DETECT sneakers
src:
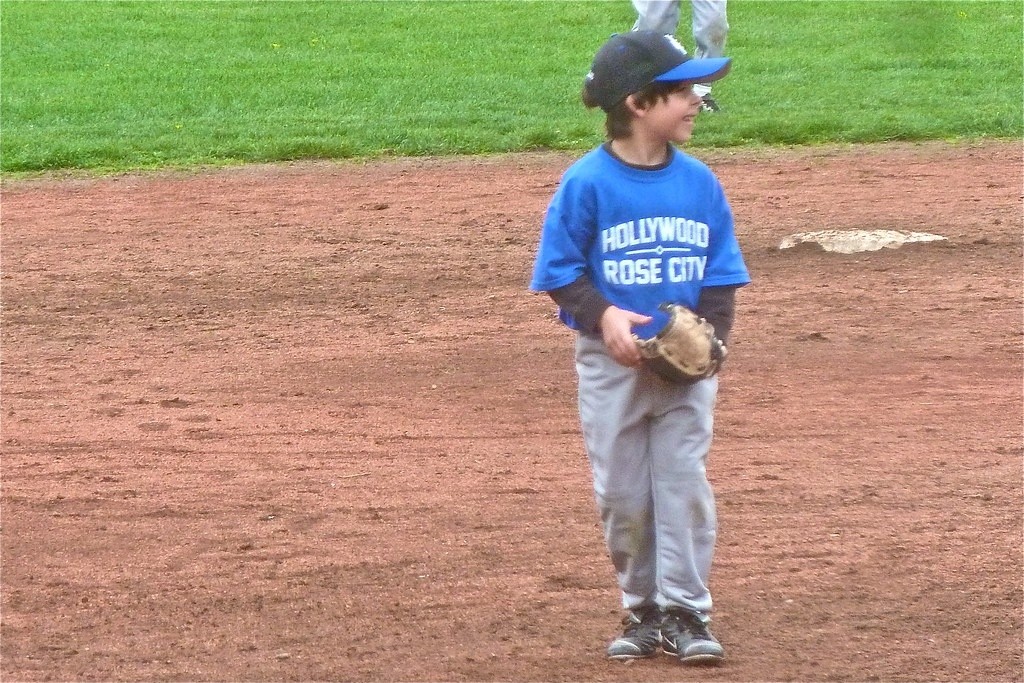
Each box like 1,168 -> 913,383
661,610 -> 725,663
582,88 -> 600,107
606,606 -> 662,660
700,93 -> 721,112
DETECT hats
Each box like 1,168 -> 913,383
586,31 -> 732,113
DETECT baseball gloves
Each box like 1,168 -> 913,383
633,303 -> 724,388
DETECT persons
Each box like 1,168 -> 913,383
531,32 -> 753,663
632,0 -> 729,113
